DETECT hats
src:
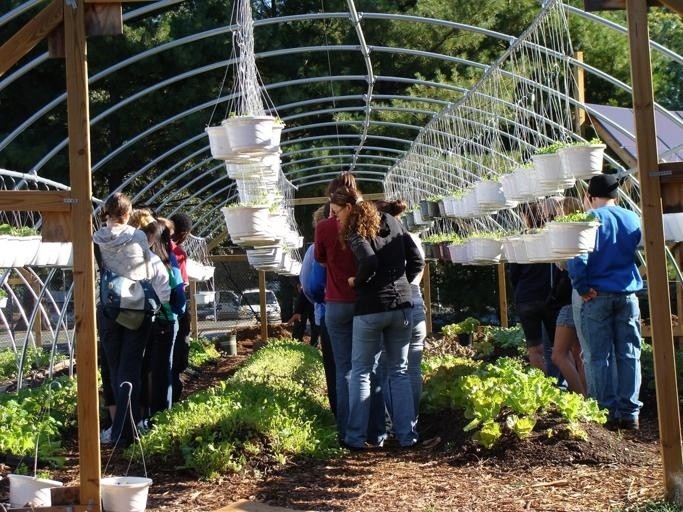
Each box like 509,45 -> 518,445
589,174 -> 618,200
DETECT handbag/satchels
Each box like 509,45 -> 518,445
100,265 -> 157,332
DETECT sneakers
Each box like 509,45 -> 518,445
100,425 -> 112,445
603,415 -> 639,430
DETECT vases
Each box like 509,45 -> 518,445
101,477 -> 152,512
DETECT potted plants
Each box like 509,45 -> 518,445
205,113 -> 304,278
7,469 -> 63,509
0,224 -> 216,282
401,140 -> 607,267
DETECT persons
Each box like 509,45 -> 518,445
506,171 -> 645,431
286,173 -> 444,452
91,190 -> 195,452
